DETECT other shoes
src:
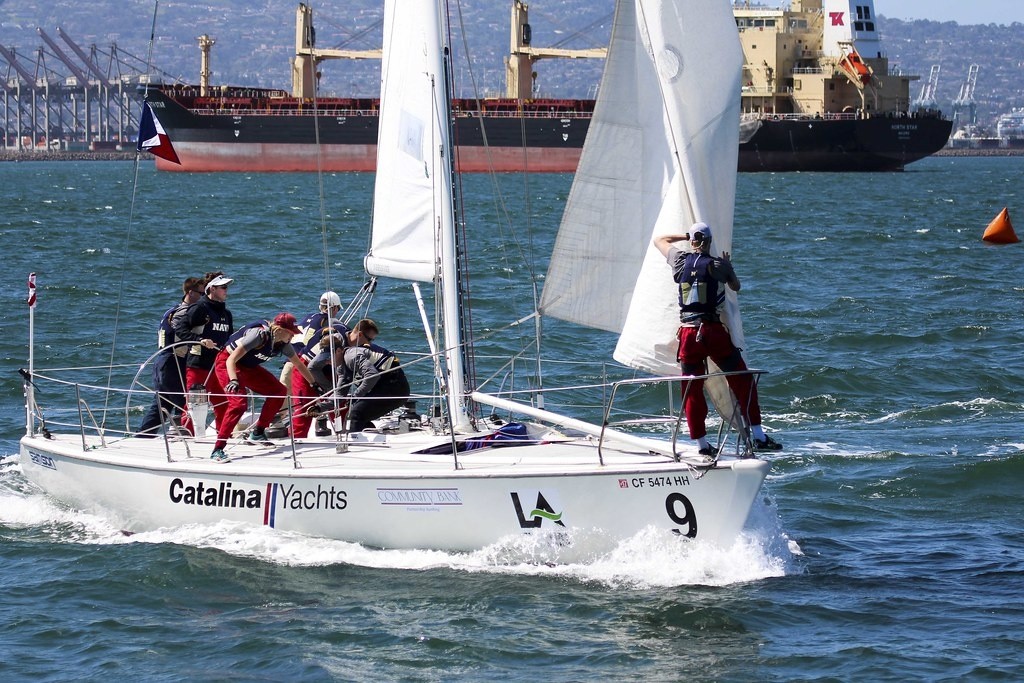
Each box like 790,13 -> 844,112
315,418 -> 331,435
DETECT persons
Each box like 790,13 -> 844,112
210,314 -> 325,463
280,292 -> 342,416
288,319 -> 380,438
136,278 -> 205,437
307,336 -> 409,432
175,270 -> 233,435
655,221 -> 782,456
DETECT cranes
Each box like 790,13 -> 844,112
949,63 -> 981,138
911,64 -> 943,113
0,24 -> 190,150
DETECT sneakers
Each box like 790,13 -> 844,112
248,433 -> 276,449
699,442 -> 721,456
753,434 -> 783,451
210,450 -> 230,463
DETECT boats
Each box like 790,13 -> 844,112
149,0 -> 958,174
841,53 -> 871,85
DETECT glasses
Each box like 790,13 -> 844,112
216,284 -> 227,289
190,289 -> 205,297
360,330 -> 374,342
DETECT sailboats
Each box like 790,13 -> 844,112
16,1 -> 778,564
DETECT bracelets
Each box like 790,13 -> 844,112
686,233 -> 689,240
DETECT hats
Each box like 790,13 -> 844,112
274,314 -> 303,334
205,275 -> 234,295
688,223 -> 712,240
320,291 -> 343,309
317,345 -> 340,361
